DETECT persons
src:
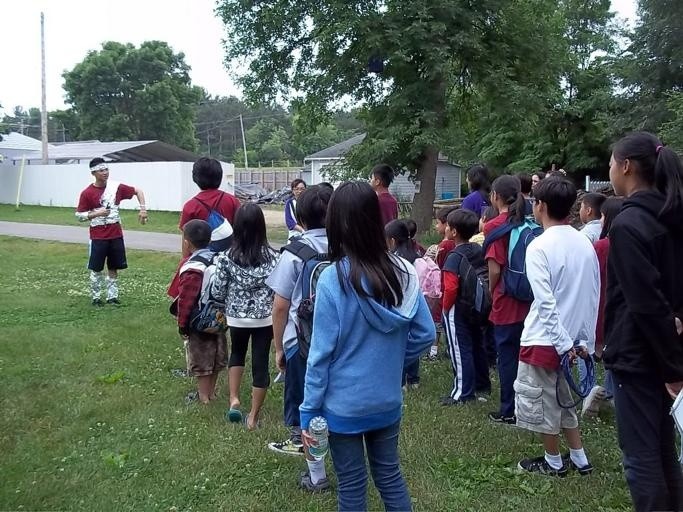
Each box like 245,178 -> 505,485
603,131 -> 683,512
366,165 -> 628,479
75,158 -> 147,306
168,158 -> 335,491
298,181 -> 436,511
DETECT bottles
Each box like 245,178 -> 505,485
308,417 -> 329,462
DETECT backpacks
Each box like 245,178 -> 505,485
190,252 -> 229,336
482,215 -> 544,302
194,193 -> 235,251
280,241 -> 334,360
411,256 -> 442,315
443,242 -> 497,315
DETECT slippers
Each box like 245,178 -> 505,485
230,409 -> 242,423
244,414 -> 259,432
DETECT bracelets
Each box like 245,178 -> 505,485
140,205 -> 145,207
141,211 -> 147,213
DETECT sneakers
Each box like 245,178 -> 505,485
267,438 -> 305,456
107,298 -> 121,307
489,412 -> 516,427
92,299 -> 104,307
439,397 -> 475,407
420,353 -> 439,364
297,470 -> 331,496
518,455 -> 568,480
562,452 -> 594,477
